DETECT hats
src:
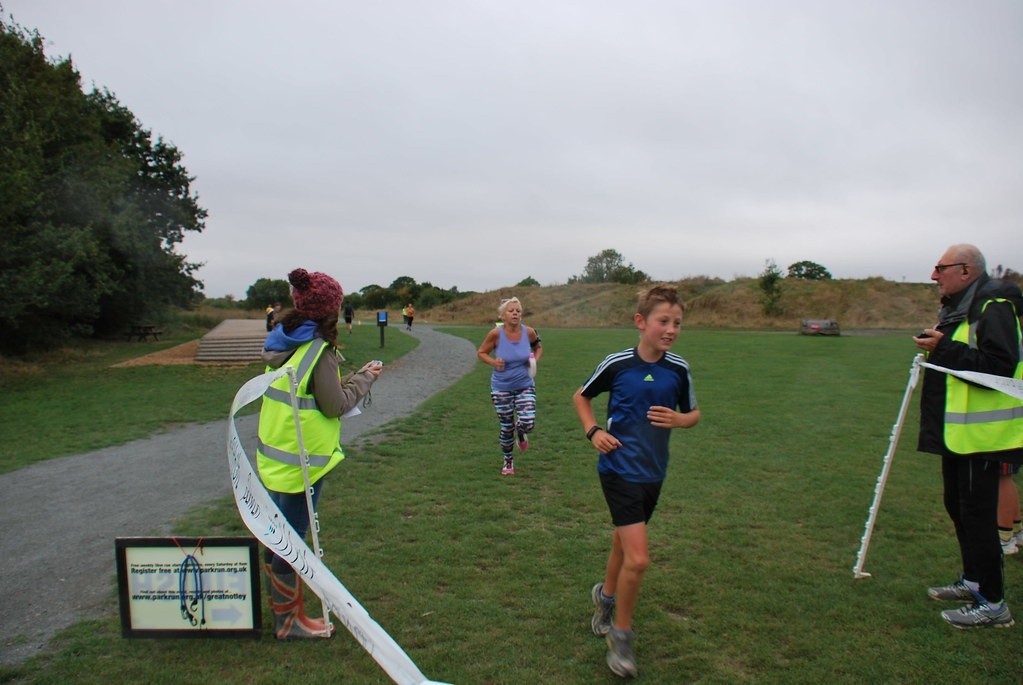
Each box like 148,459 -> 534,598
287,267 -> 343,319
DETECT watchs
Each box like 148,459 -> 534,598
586,425 -> 602,441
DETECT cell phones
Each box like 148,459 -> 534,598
368,360 -> 382,368
917,333 -> 932,339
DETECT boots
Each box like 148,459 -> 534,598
264,562 -> 336,639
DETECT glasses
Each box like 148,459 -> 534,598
935,263 -> 967,273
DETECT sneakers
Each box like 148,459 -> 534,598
1012,528 -> 1023,546
591,582 -> 613,637
515,423 -> 529,451
501,458 -> 514,475
941,592 -> 1016,630
927,571 -> 979,604
606,619 -> 638,679
998,536 -> 1019,556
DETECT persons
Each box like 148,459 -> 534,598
913,243 -> 1023,630
256,267 -> 383,640
267,302 -> 281,331
343,300 -> 354,334
403,303 -> 414,330
998,271 -> 1023,555
478,297 -> 543,475
573,286 -> 700,677
